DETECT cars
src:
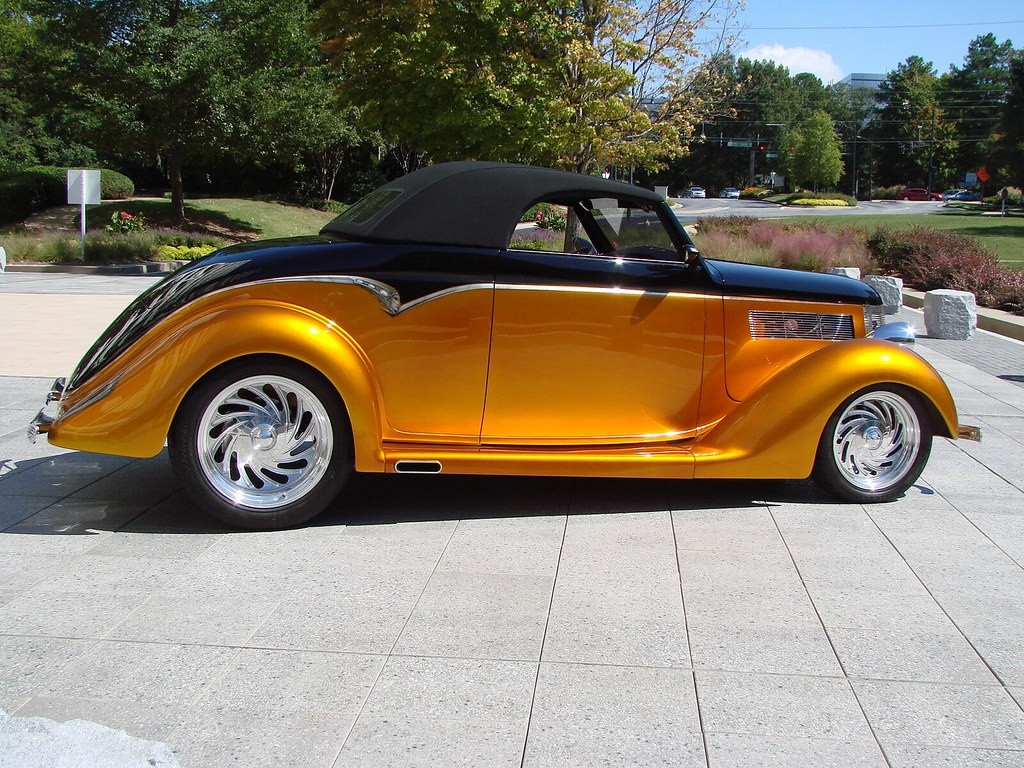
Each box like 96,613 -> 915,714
897,187 -> 943,201
943,190 -> 981,202
39,160 -> 986,531
688,183 -> 705,196
719,187 -> 738,200
